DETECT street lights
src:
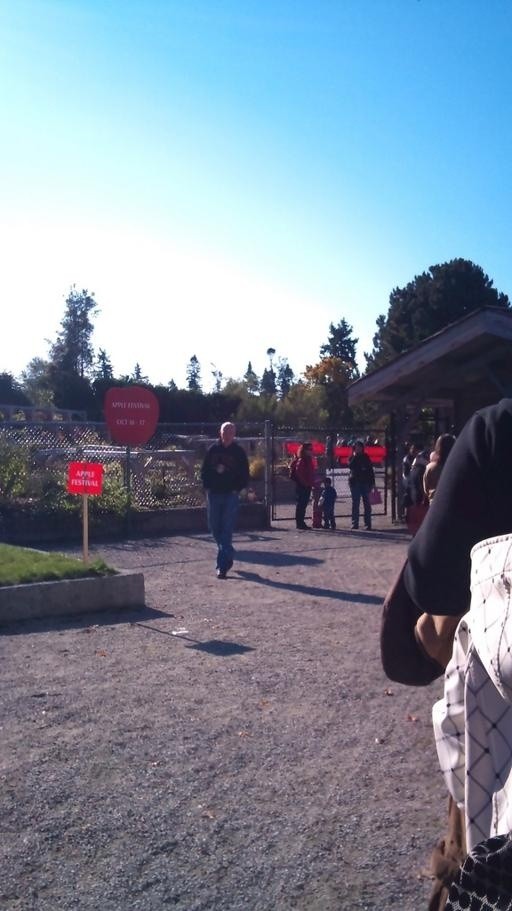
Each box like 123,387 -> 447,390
190,355 -> 199,392
267,348 -> 277,400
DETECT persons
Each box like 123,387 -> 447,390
430,532 -> 512,911
201,421 -> 249,577
348,442 -> 374,529
381,396 -> 512,911
319,478 -> 337,530
295,443 -> 314,529
325,434 -> 380,471
402,434 -> 456,535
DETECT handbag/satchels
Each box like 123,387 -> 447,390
406,496 -> 429,534
288,457 -> 304,481
368,486 -> 382,504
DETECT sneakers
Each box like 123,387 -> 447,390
216,546 -> 235,578
296,520 -> 371,530
